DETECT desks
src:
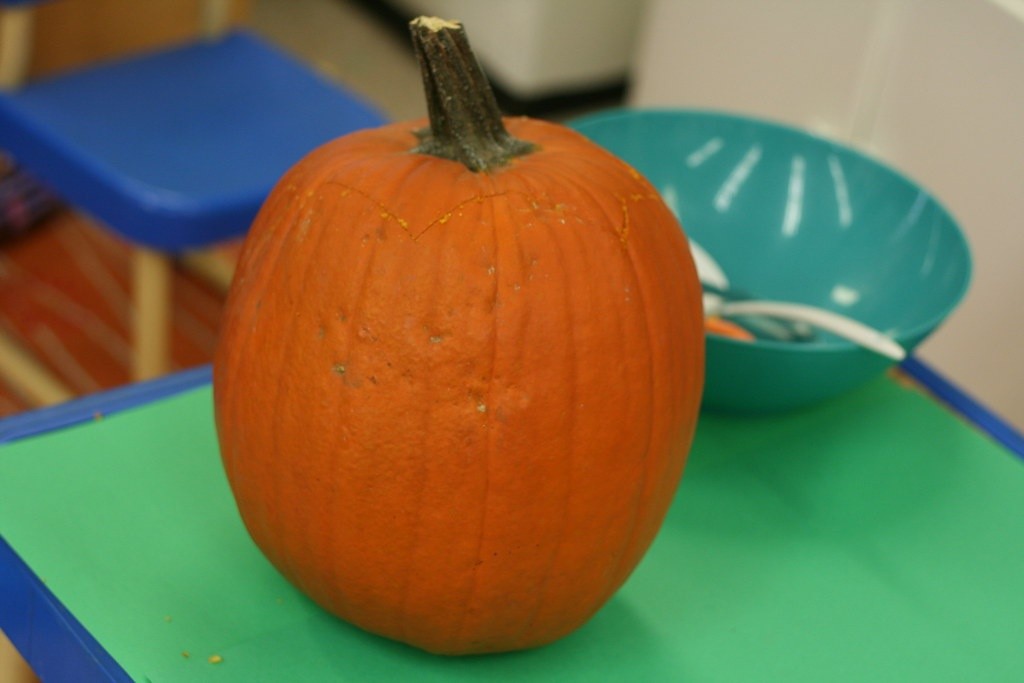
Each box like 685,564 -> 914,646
0,358 -> 1024,683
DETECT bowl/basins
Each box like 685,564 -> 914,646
563,105 -> 976,418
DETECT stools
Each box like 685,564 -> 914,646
0,29 -> 395,405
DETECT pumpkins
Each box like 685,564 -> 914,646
213,14 -> 705,656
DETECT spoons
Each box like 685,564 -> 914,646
680,230 -> 906,367
